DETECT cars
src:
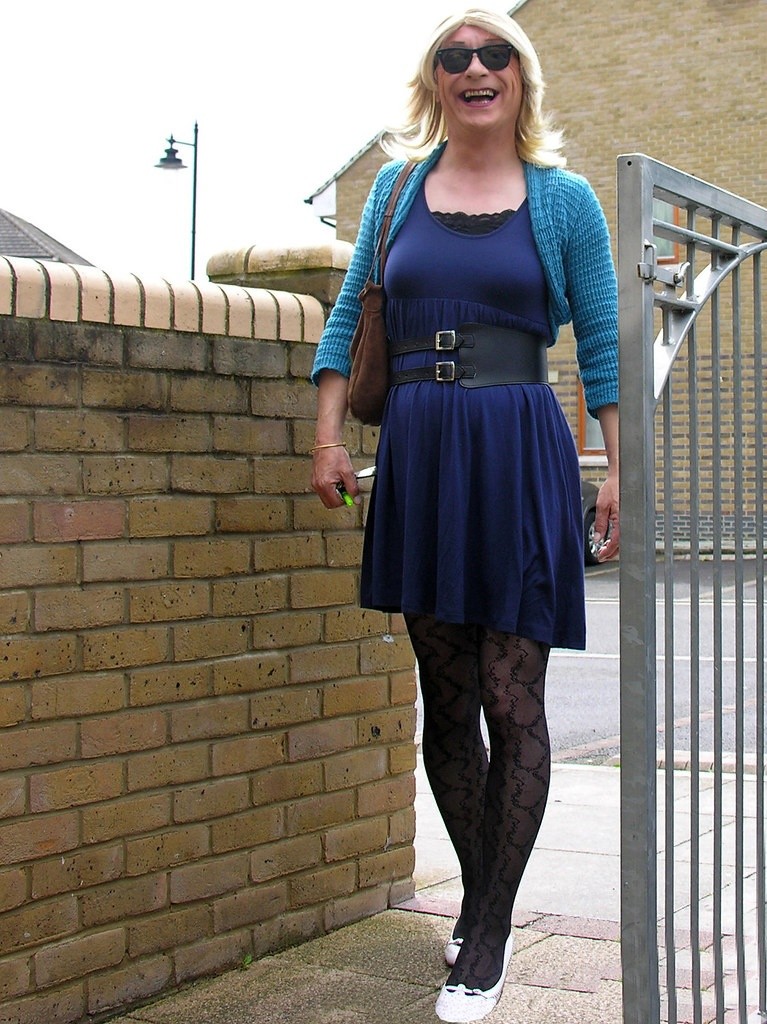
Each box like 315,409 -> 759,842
581,478 -> 612,567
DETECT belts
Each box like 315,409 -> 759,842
386,322 -> 550,389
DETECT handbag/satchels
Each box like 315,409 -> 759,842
348,161 -> 419,426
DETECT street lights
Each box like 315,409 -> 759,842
151,120 -> 200,280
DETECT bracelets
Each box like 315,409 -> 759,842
310,443 -> 346,453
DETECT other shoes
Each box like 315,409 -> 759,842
435,927 -> 513,1022
444,915 -> 466,966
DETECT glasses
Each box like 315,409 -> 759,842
434,44 -> 518,74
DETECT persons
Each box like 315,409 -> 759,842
306,11 -> 621,1024
